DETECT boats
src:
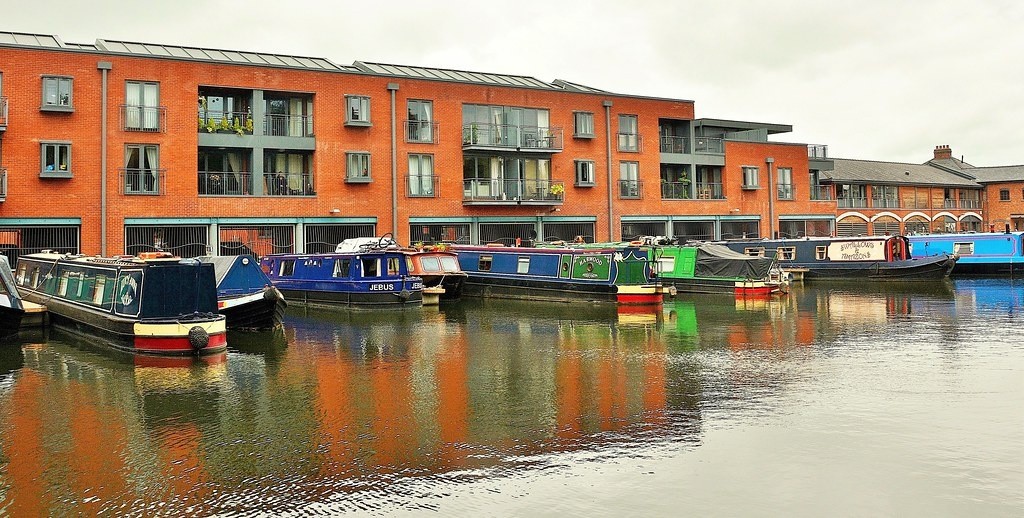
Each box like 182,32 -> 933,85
0,254 -> 26,330
434,231 -> 1024,295
13,247 -> 228,356
188,254 -> 288,334
257,247 -> 424,306
366,243 -> 469,297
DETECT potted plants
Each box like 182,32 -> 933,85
466,132 -> 471,144
469,121 -> 479,144
550,184 -> 564,199
216,116 -> 233,134
198,117 -> 216,133
233,117 -> 253,136
463,138 -> 468,145
414,240 -> 424,252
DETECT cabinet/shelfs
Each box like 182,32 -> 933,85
697,189 -> 711,199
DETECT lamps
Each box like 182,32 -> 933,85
330,208 -> 340,213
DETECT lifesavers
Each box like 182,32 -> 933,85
139,252 -> 173,260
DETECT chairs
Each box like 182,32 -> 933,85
286,178 -> 300,195
528,186 -> 540,200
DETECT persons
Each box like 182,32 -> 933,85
276,172 -> 290,195
574,234 -> 585,244
529,229 -> 537,241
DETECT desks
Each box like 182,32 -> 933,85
535,187 -> 547,199
544,136 -> 557,148
